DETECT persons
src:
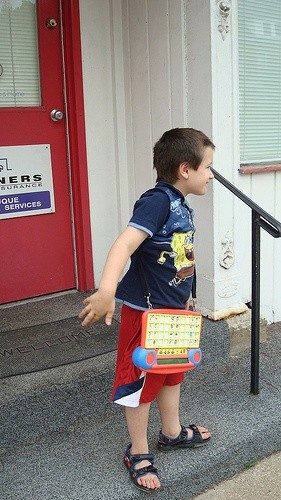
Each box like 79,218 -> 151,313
78,127 -> 215,492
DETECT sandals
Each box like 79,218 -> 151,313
124,442 -> 159,493
157,423 -> 210,452
